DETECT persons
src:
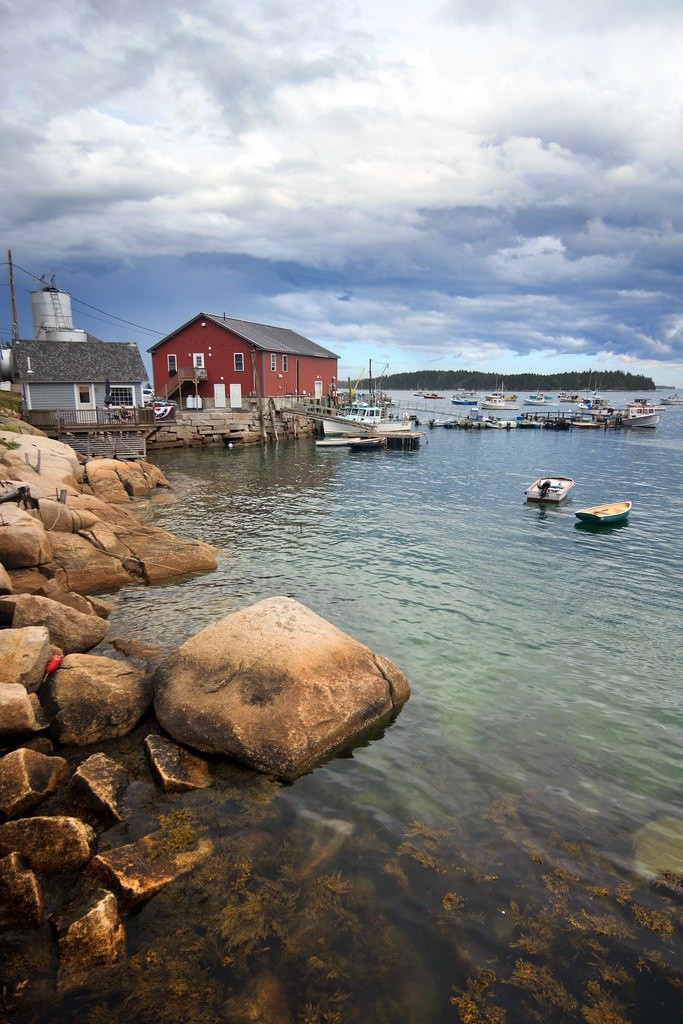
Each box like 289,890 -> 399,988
526,412 -> 533,423
617,410 -> 622,417
102,404 -> 129,425
335,394 -> 341,409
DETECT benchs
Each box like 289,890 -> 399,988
548,488 -> 559,492
593,510 -> 610,515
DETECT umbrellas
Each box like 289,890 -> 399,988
104,377 -> 113,421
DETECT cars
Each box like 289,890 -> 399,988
143,389 -> 155,402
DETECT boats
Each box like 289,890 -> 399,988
451,392 -> 483,404
557,390 -> 582,402
414,391 -> 428,396
321,406 -> 413,437
522,391 -> 560,406
479,397 -> 520,410
485,376 -> 518,401
524,476 -> 575,503
414,395 -> 667,431
574,501 -> 632,524
424,393 -> 447,400
660,393 -> 683,406
347,438 -> 382,449
578,392 -> 615,415
